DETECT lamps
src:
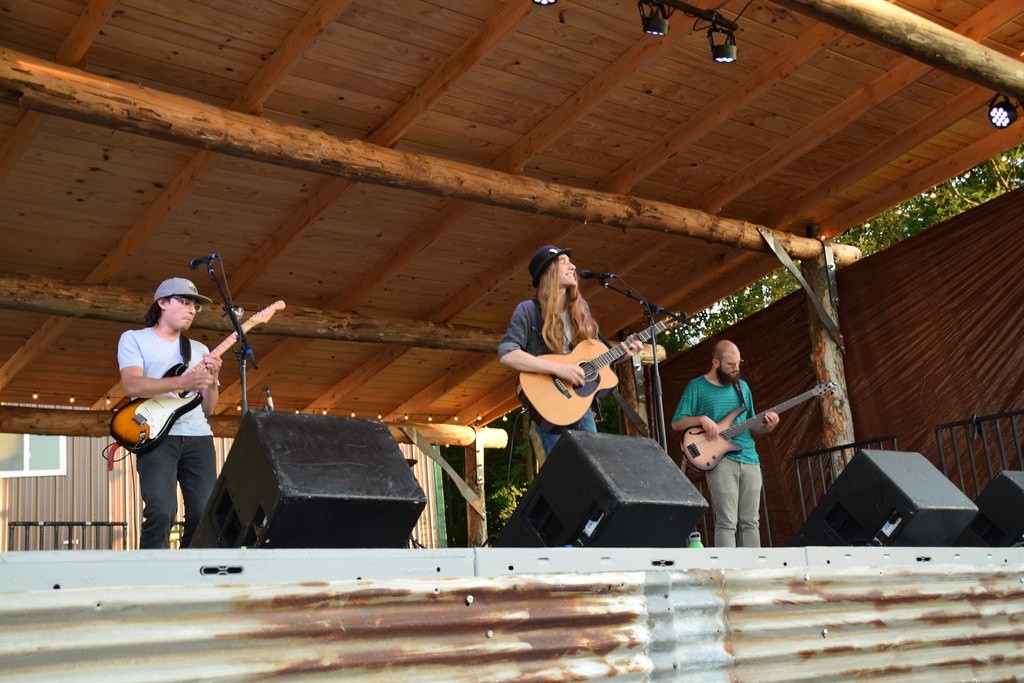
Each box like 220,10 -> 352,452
707,27 -> 739,65
986,95 -> 1022,130
637,4 -> 673,37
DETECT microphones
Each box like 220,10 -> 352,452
189,253 -> 218,270
580,270 -> 618,280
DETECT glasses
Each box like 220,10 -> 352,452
718,360 -> 744,368
170,295 -> 202,313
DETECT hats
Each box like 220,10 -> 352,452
153,277 -> 213,305
528,245 -> 571,288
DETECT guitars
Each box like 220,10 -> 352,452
109,300 -> 287,455
516,310 -> 687,433
680,379 -> 837,472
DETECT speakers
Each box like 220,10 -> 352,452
788,448 -> 979,548
189,408 -> 427,549
492,429 -> 709,549
952,470 -> 1024,547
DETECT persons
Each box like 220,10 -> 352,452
117,277 -> 223,549
498,245 -> 643,459
672,339 -> 780,548
